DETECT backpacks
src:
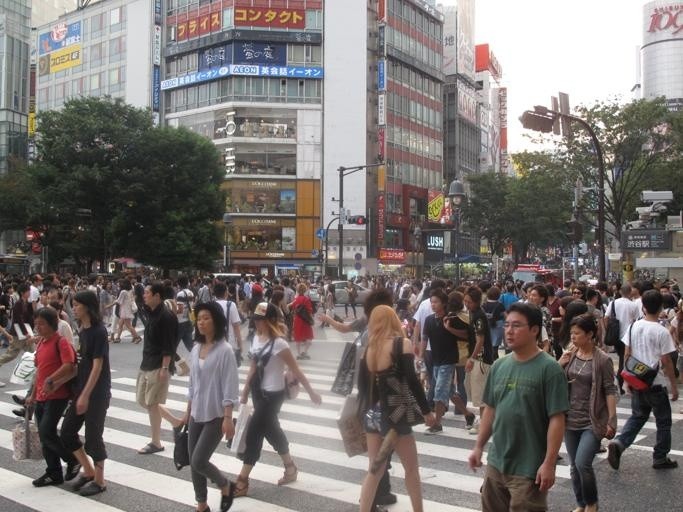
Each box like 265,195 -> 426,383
484,301 -> 501,328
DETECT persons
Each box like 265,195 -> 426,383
0,270 -> 682,511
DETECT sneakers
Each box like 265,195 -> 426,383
607,442 -> 622,470
108,335 -> 142,344
424,424 -> 443,435
12,395 -> 25,405
65,463 -> 82,481
32,473 -> 64,486
296,350 -> 311,360
374,495 -> 395,504
465,413 -> 482,434
652,458 -> 677,469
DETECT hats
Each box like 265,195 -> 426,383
254,302 -> 278,317
255,274 -> 265,280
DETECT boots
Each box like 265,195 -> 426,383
177,358 -> 190,376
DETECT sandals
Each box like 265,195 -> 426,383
277,462 -> 298,485
233,474 -> 249,497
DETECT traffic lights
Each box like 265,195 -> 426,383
349,215 -> 365,225
564,220 -> 582,242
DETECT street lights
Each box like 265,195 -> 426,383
521,107 -> 606,281
414,215 -> 459,285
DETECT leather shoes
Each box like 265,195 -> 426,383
220,480 -> 235,512
12,407 -> 32,420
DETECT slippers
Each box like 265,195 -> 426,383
72,473 -> 95,490
174,421 -> 185,438
138,444 -> 165,454
79,480 -> 107,496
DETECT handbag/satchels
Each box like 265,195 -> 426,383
131,302 -> 138,313
605,319 -> 620,345
378,369 -> 425,426
230,403 -> 253,454
232,347 -> 241,368
189,309 -> 195,323
620,353 -> 656,392
12,422 -> 45,461
283,370 -> 299,402
174,432 -> 190,471
329,343 -> 368,459
296,304 -> 315,324
10,352 -> 36,385
